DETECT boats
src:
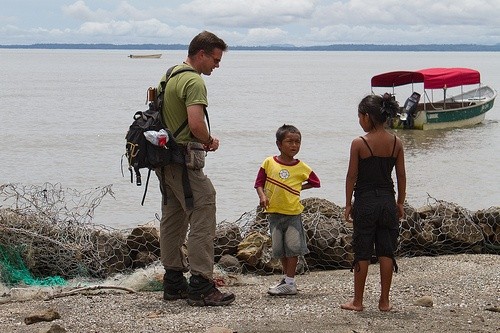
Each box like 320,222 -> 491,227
371,67 -> 496,131
129,54 -> 162,58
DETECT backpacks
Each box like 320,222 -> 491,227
119,65 -> 211,186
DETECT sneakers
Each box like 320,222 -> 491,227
268,281 -> 298,295
271,279 -> 297,288
187,287 -> 235,306
163,276 -> 188,300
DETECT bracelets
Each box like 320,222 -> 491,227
207,137 -> 213,144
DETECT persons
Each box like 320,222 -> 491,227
339,93 -> 407,311
152,32 -> 235,306
254,124 -> 320,295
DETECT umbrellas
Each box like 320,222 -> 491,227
145,86 -> 157,111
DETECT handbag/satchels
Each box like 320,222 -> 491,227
185,142 -> 205,170
